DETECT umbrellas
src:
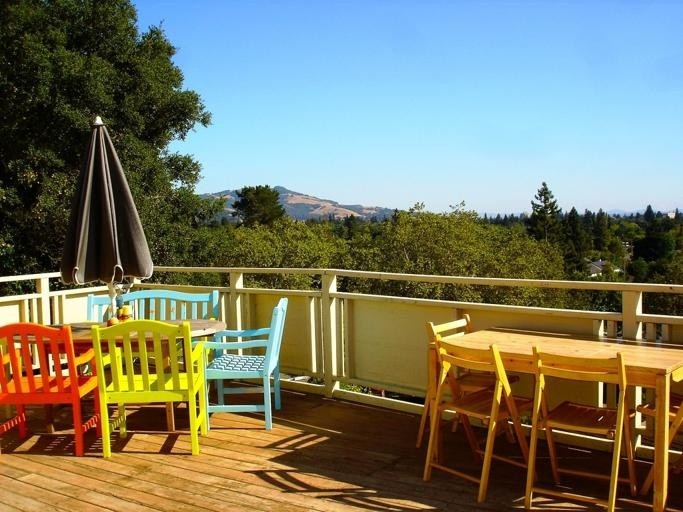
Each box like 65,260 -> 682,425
60,113 -> 154,323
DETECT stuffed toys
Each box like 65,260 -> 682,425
102,296 -> 135,327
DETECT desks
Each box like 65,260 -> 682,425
433,325 -> 683,467
5,318 -> 228,376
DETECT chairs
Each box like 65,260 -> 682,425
421,332 -> 540,506
523,345 -> 638,511
0,321 -> 102,459
86,290 -> 226,365
414,314 -> 519,449
635,399 -> 683,496
88,317 -> 207,457
192,288 -> 291,443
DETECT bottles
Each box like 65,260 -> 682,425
114,288 -> 124,308
